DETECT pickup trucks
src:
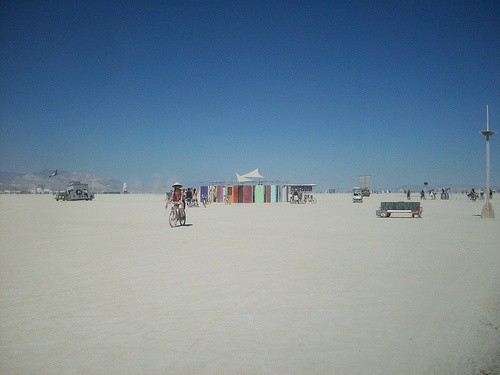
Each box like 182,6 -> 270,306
362,189 -> 370,197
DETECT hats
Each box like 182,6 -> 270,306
172,182 -> 182,188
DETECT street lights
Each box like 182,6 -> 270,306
481,104 -> 495,219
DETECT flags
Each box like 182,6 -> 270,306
49,170 -> 57,177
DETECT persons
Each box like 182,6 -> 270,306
169,183 -> 186,210
430,189 -> 435,199
407,190 -> 411,199
290,188 -> 302,204
186,188 -> 198,207
420,190 -> 424,199
471,187 -> 475,198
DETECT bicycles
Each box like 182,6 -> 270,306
202,197 -> 207,207
166,202 -> 186,228
302,194 -> 316,204
